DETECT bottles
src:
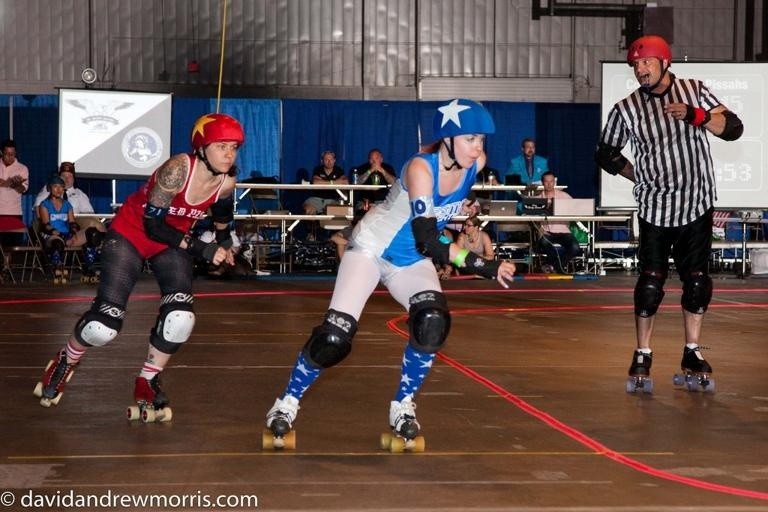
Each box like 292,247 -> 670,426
372,169 -> 380,185
487,170 -> 495,186
351,168 -> 359,185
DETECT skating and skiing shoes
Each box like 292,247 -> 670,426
126,371 -> 173,423
33,347 -> 80,408
51,260 -> 69,285
379,395 -> 425,453
626,350 -> 653,394
673,346 -> 715,393
80,264 -> 101,285
262,395 -> 301,450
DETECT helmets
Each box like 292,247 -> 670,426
191,113 -> 244,148
627,35 -> 672,67
433,99 -> 496,142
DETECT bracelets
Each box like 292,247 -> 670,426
692,108 -> 705,126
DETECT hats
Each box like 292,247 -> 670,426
59,162 -> 76,180
46,176 -> 65,192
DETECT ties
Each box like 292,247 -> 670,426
63,189 -> 67,201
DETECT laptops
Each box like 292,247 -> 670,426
522,197 -> 548,214
488,200 -> 518,216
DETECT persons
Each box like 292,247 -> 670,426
32,163 -> 97,213
43,112 -> 246,402
595,34 -> 744,376
0,142 -> 30,277
201,139 -> 579,274
39,175 -> 101,277
267,98 -> 517,438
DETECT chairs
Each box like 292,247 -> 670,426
0,176 -> 534,284
